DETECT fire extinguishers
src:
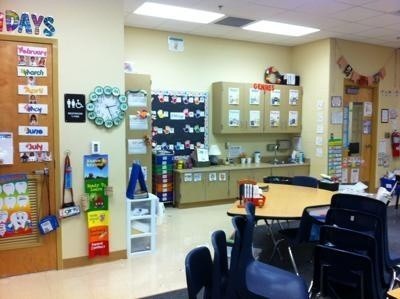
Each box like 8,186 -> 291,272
391,130 -> 400,158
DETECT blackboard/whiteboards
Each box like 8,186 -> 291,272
151,92 -> 208,159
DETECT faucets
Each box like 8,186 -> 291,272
274,144 -> 279,160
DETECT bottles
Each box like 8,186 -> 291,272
254,152 -> 261,164
299,152 -> 304,164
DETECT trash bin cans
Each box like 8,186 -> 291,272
380,177 -> 400,193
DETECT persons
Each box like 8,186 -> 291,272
19,57 -> 25,64
30,114 -> 38,125
29,56 -> 37,65
29,76 -> 34,84
30,95 -> 36,103
19,150 -> 52,163
40,57 -> 45,65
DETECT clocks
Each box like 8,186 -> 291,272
85,84 -> 128,129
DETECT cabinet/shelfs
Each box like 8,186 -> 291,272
229,168 -> 271,203
212,81 -> 303,135
125,72 -> 157,258
172,171 -> 229,208
270,166 -> 310,178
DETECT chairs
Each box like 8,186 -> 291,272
185,193 -> 400,298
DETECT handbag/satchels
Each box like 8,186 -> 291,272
58,202 -> 81,219
38,214 -> 60,235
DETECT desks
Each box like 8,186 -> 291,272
227,181 -> 335,260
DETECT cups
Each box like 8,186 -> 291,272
241,158 -> 252,164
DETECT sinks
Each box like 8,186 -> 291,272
265,160 -> 296,165
